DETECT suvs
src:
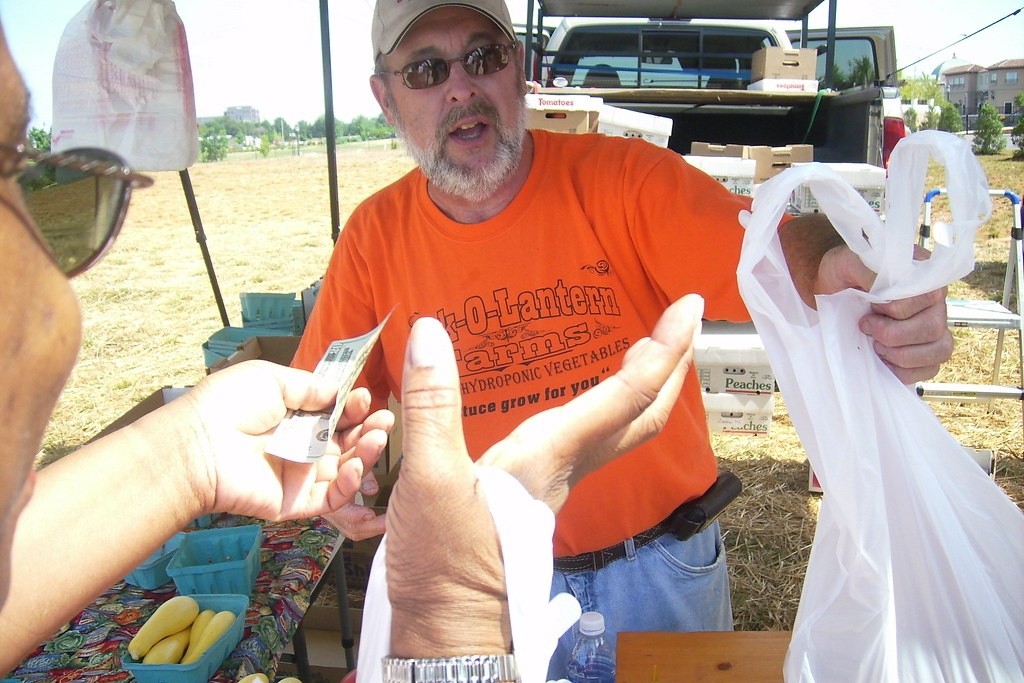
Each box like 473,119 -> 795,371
506,15 -> 910,184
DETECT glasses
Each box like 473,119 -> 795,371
0,146 -> 157,279
378,42 -> 516,90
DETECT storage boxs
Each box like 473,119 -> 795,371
165,524 -> 262,596
748,46 -> 819,94
598,105 -> 673,148
689,142 -> 749,158
681,155 -> 757,197
693,321 -> 775,396
123,532 -> 185,590
748,143 -> 814,185
119,593 -> 249,683
789,162 -> 887,214
524,92 -> 603,135
701,391 -> 775,435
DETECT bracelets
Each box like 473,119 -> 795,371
380,653 -> 517,683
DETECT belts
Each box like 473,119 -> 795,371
553,518 -> 672,573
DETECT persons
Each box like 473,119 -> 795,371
0,24 -> 703,683
286,0 -> 955,683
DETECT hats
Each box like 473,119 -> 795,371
372,0 -> 517,63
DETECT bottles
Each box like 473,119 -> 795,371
566,611 -> 616,683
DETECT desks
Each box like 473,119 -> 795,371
0,513 -> 355,683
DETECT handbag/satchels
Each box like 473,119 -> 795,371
733,128 -> 1024,682
